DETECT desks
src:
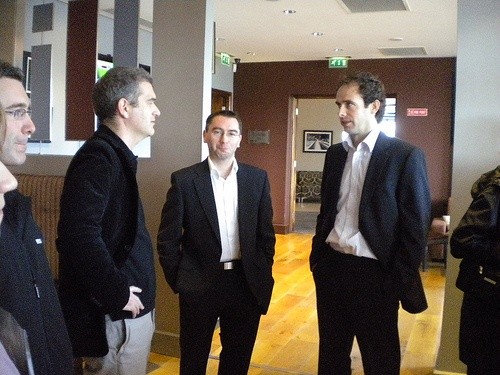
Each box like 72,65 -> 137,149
422,232 -> 448,272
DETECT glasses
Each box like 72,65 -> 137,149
3,108 -> 34,119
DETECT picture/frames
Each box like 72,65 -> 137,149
302,130 -> 333,153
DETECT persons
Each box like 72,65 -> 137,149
309,71 -> 434,375
449,165 -> 500,375
0,60 -> 76,375
54,66 -> 161,374
156,110 -> 278,375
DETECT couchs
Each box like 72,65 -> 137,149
296,170 -> 323,203
